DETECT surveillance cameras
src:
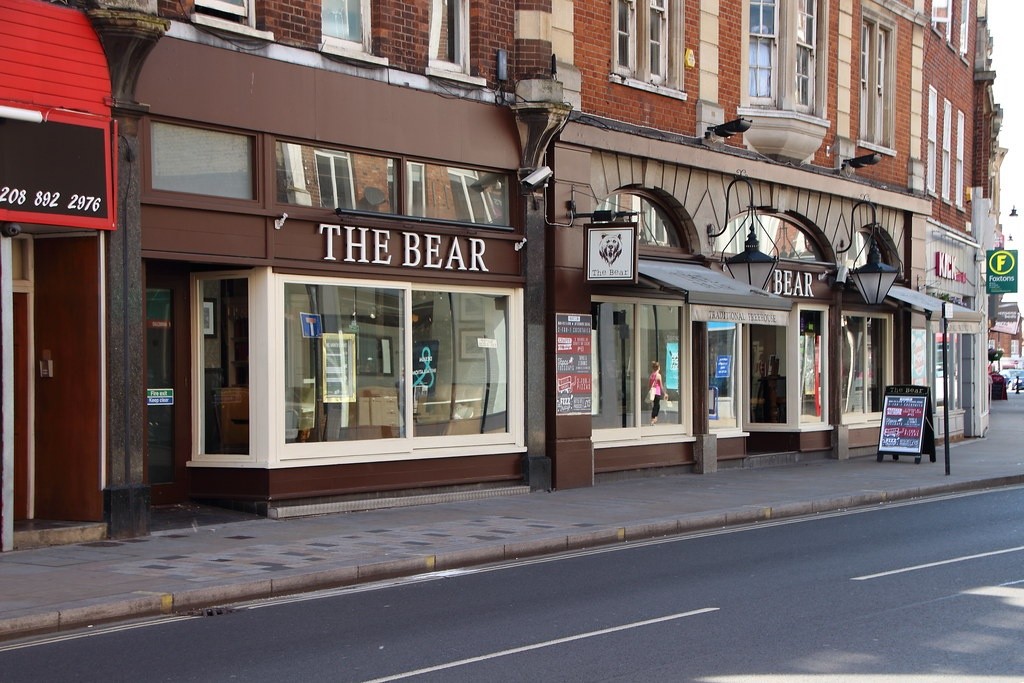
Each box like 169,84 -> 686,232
520,166 -> 552,189
836,266 -> 849,284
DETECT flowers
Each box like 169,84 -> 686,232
988,342 -> 1005,360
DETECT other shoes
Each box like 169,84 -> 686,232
650,416 -> 657,426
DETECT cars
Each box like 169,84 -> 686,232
998,369 -> 1024,392
935,342 -> 950,379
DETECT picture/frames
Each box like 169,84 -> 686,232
459,327 -> 486,362
450,382 -> 486,420
202,297 -> 218,339
458,293 -> 485,323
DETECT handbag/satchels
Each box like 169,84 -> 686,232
645,387 -> 656,403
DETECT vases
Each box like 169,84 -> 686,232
988,353 -> 1002,361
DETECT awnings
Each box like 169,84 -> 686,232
886,285 -> 982,334
636,260 -> 791,326
990,302 -> 1021,336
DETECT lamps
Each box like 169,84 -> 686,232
994,230 -> 1014,242
987,202 -> 1019,217
840,152 -> 885,169
833,193 -> 901,307
704,117 -> 754,139
707,169 -> 780,291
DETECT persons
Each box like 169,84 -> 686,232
649,361 -> 668,426
